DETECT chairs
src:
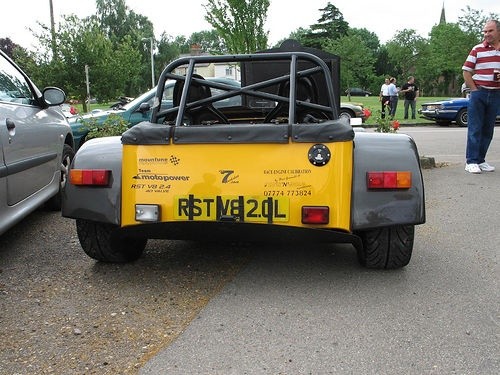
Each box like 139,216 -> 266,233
162,74 -> 230,125
263,72 -> 329,124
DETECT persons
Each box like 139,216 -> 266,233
461,19 -> 500,173
388,77 -> 403,120
400,76 -> 419,119
379,78 -> 391,119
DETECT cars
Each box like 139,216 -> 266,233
84,96 -> 106,104
345,88 -> 372,97
417,93 -> 500,127
0,48 -> 76,235
67,77 -> 371,156
65,98 -> 78,104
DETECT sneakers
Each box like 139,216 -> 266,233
479,162 -> 495,172
464,163 -> 482,174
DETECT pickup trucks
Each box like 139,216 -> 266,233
62,39 -> 426,270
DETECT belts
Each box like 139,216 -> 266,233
479,88 -> 500,92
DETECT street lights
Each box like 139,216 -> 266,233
141,37 -> 155,89
